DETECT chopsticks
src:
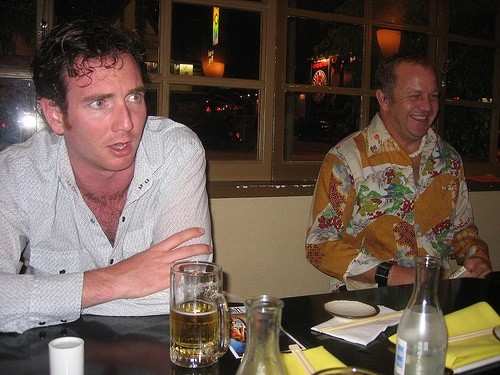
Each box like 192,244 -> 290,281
318,308 -> 405,333
448,328 -> 494,342
289,344 -> 316,375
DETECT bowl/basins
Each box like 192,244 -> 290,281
493,326 -> 500,341
323,299 -> 380,322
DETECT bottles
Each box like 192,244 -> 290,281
236,296 -> 288,375
394,256 -> 448,375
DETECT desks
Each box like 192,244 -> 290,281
0,275 -> 500,375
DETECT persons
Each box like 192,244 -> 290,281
0,18 -> 213,333
305,52 -> 492,294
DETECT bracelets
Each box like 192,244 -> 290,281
375,262 -> 393,288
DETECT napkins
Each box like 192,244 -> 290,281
312,306 -> 402,346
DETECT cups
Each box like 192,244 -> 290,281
169,261 -> 228,368
312,366 -> 378,375
49,337 -> 84,375
